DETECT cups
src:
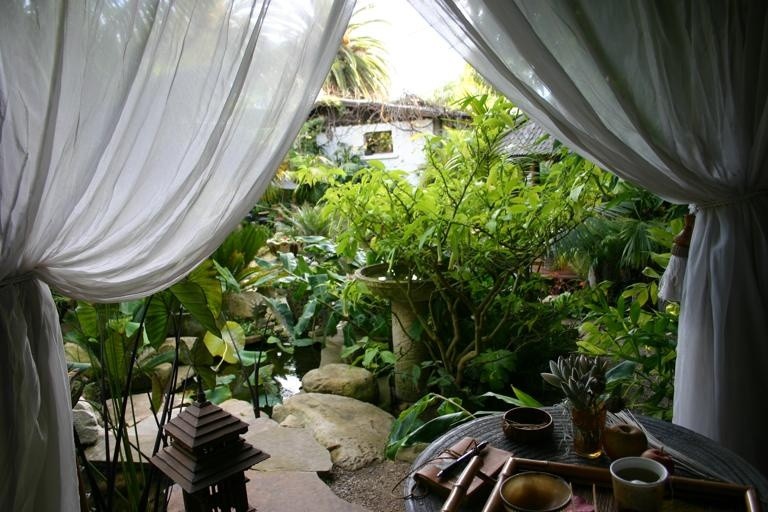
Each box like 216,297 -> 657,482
609,455 -> 669,511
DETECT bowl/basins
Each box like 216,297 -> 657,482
501,406 -> 555,444
498,470 -> 574,511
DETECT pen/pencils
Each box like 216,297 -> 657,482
437,440 -> 489,478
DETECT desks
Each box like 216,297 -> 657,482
400,407 -> 768,511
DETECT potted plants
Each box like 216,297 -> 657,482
540,349 -> 610,460
